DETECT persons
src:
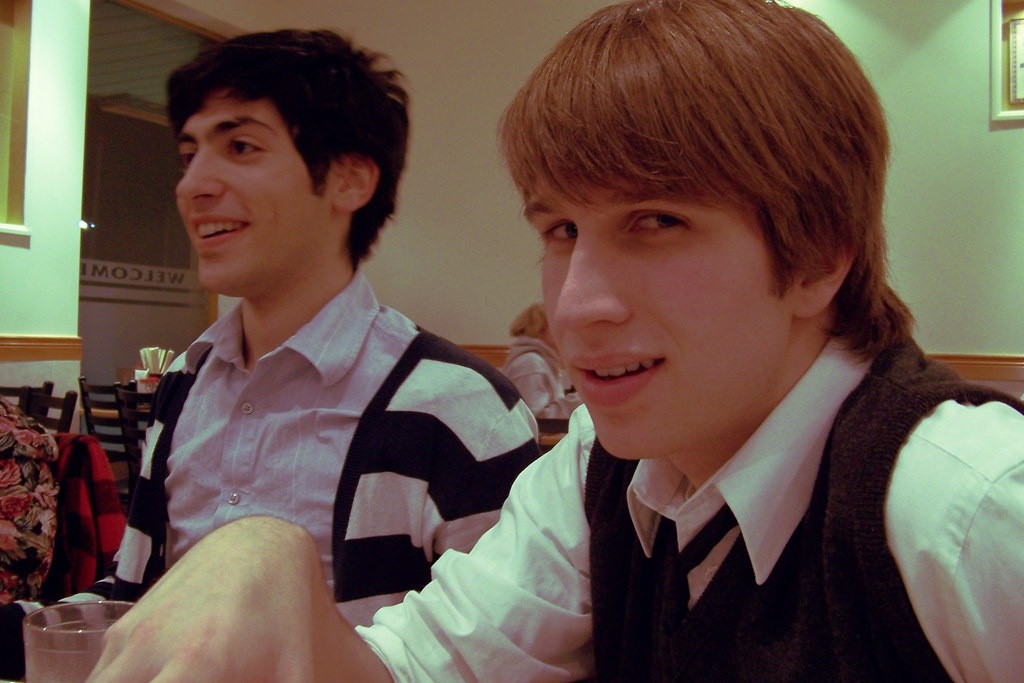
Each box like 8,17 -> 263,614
88,0 -> 1024,683
0,27 -> 546,683
504,301 -> 585,432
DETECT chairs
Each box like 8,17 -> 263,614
0,376 -> 155,602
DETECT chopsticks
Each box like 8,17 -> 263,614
139,347 -> 175,373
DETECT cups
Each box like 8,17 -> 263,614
137,374 -> 162,393
23,600 -> 135,683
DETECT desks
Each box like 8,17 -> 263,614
80,407 -> 150,419
